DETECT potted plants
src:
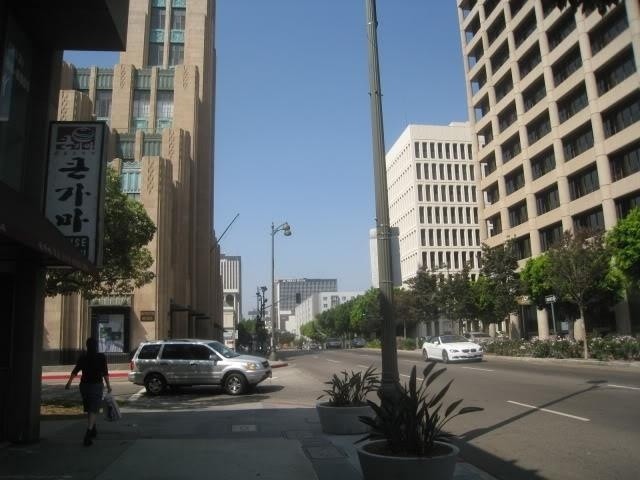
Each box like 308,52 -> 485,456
312,362 -> 380,434
355,355 -> 486,480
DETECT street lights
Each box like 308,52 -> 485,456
256,286 -> 267,355
269,220 -> 292,361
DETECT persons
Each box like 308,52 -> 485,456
64,335 -> 114,447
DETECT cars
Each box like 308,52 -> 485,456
419,334 -> 483,363
301,340 -> 323,351
275,344 -> 281,350
325,337 -> 341,349
462,331 -> 494,346
349,337 -> 367,349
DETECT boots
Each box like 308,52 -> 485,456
84,424 -> 96,446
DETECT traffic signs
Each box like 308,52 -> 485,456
296,293 -> 301,304
545,294 -> 559,305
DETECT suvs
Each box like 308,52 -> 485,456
128,338 -> 272,396
